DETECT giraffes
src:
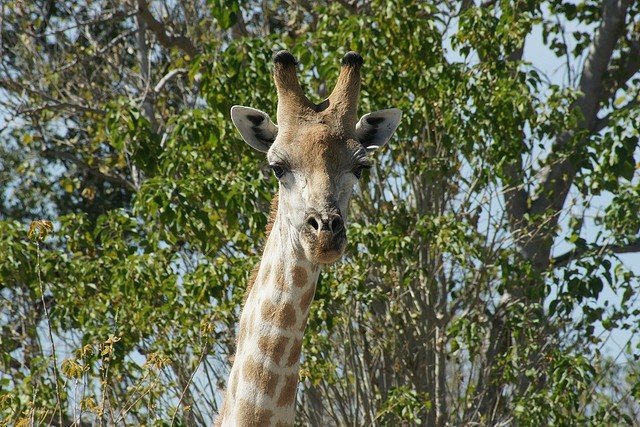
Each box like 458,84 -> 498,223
215,49 -> 403,427
